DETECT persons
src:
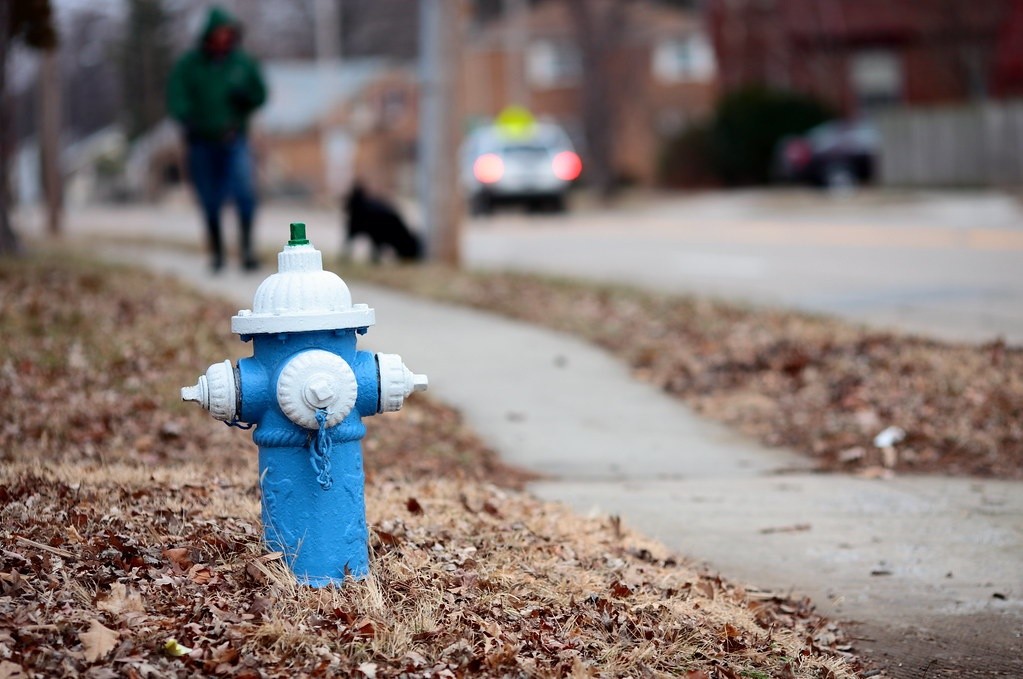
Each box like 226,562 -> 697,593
166,7 -> 268,271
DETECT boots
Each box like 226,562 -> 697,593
240,214 -> 258,270
205,216 -> 223,269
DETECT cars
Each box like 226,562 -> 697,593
661,95 -> 870,186
454,105 -> 583,221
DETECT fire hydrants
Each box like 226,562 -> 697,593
179,220 -> 432,593
